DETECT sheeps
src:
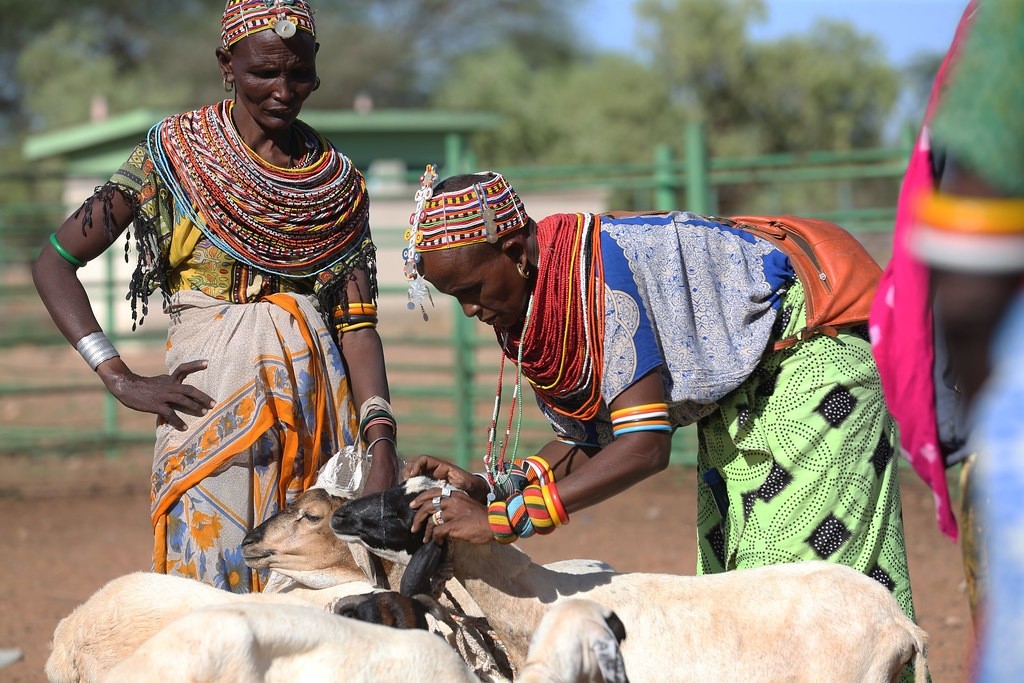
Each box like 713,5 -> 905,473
44,571 -> 630,683
329,473 -> 931,683
239,487 -> 614,619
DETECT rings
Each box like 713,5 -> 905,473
442,487 -> 451,496
432,496 -> 441,511
432,510 -> 444,526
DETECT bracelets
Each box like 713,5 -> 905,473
488,482 -> 569,545
76,331 -> 121,371
473,456 -> 555,501
360,411 -> 397,468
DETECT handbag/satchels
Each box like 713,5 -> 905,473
726,211 -> 889,338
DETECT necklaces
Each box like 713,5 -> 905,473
286,123 -> 319,170
482,256 -> 541,503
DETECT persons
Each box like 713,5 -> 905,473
29,0 -> 398,593
876,0 -> 1024,683
403,171 -> 932,683
